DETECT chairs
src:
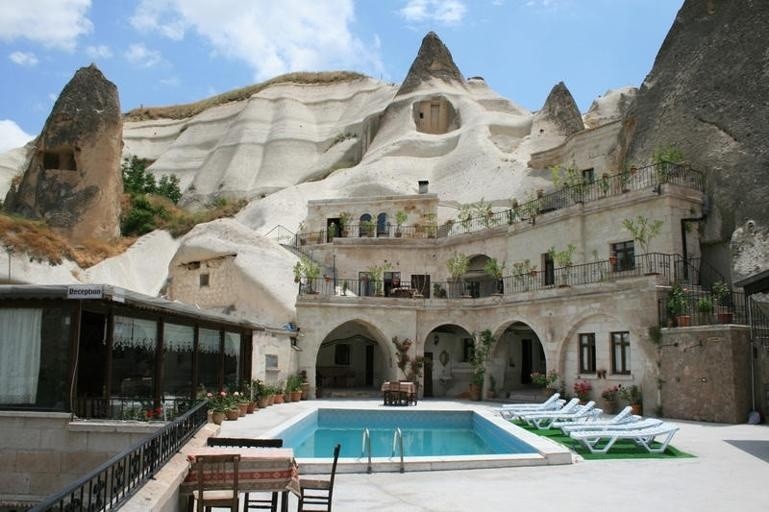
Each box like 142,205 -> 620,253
381,379 -> 419,406
207,437 -> 283,512
195,454 -> 240,512
297,443 -> 341,512
500,393 -> 680,454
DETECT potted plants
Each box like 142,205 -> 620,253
665,279 -> 734,327
292,148 -> 661,299
468,329 -> 496,400
207,371 -> 310,425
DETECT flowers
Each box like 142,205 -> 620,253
531,371 -> 641,406
